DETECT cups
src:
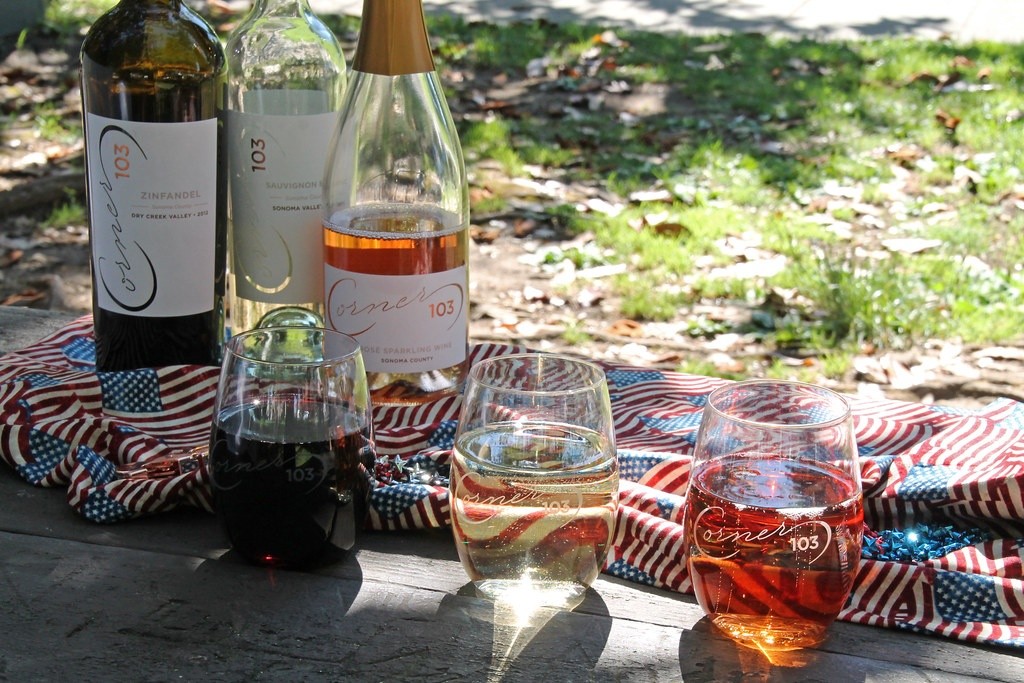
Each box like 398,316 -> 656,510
210,325 -> 377,572
446,352 -> 621,612
681,378 -> 865,654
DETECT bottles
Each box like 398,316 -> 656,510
319,0 -> 474,407
76,1 -> 231,378
226,0 -> 348,381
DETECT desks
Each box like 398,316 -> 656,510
0,304 -> 1024,683
